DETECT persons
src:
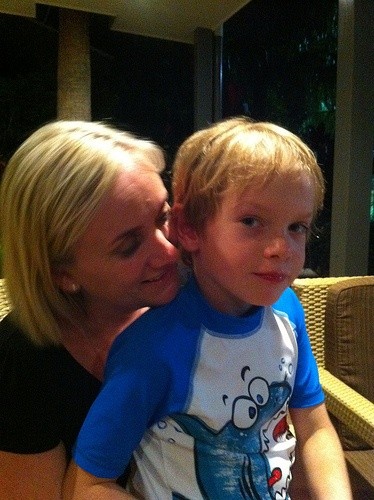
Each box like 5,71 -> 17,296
0,118 -> 181,500
65,114 -> 355,500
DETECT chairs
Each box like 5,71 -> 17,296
290,276 -> 374,500
